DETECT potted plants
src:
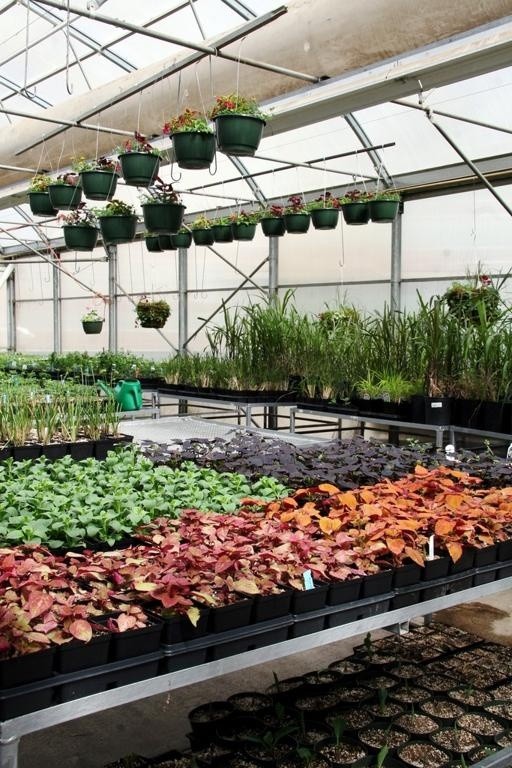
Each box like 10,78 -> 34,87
0,266 -> 512,767
310,191 -> 338,229
99,198 -> 140,244
70,154 -> 121,201
117,131 -> 170,188
47,171 -> 83,210
339,189 -> 370,225
366,187 -> 400,223
282,194 -> 311,233
209,94 -> 274,158
160,109 -> 218,171
140,183 -> 186,234
28,174 -> 59,218
145,210 -> 258,253
59,202 -> 99,252
255,202 -> 286,236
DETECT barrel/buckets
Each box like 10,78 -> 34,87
83,322 -> 103,336
